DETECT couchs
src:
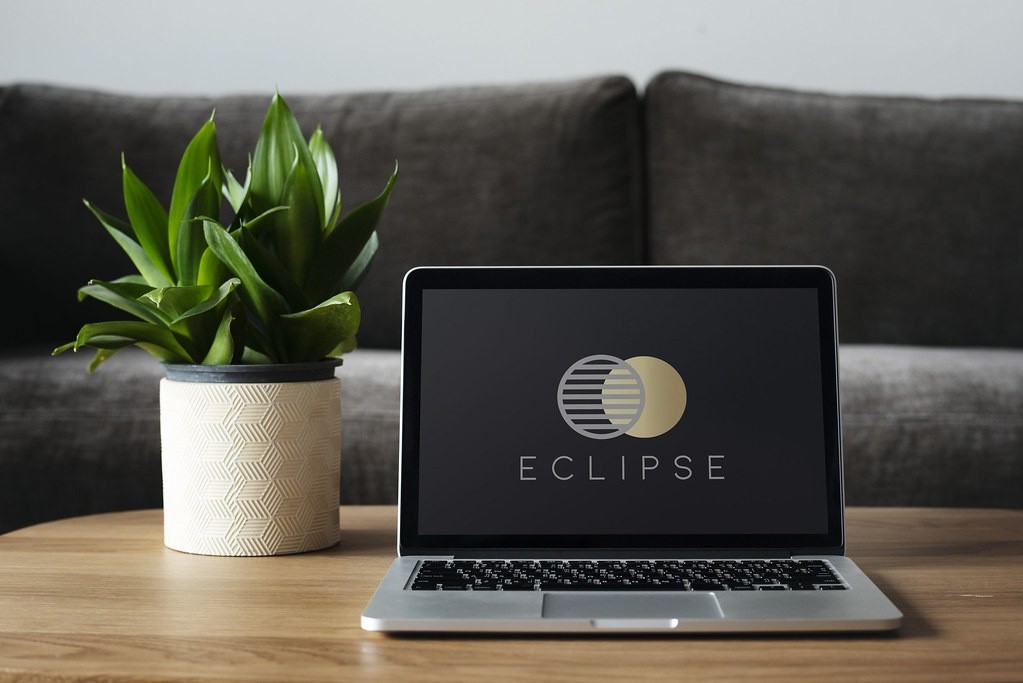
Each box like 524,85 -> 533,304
0,70 -> 1023,541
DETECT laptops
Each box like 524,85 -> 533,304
360,264 -> 905,634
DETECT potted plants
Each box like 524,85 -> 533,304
52,85 -> 398,556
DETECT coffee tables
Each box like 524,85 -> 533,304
0,506 -> 1020,683
